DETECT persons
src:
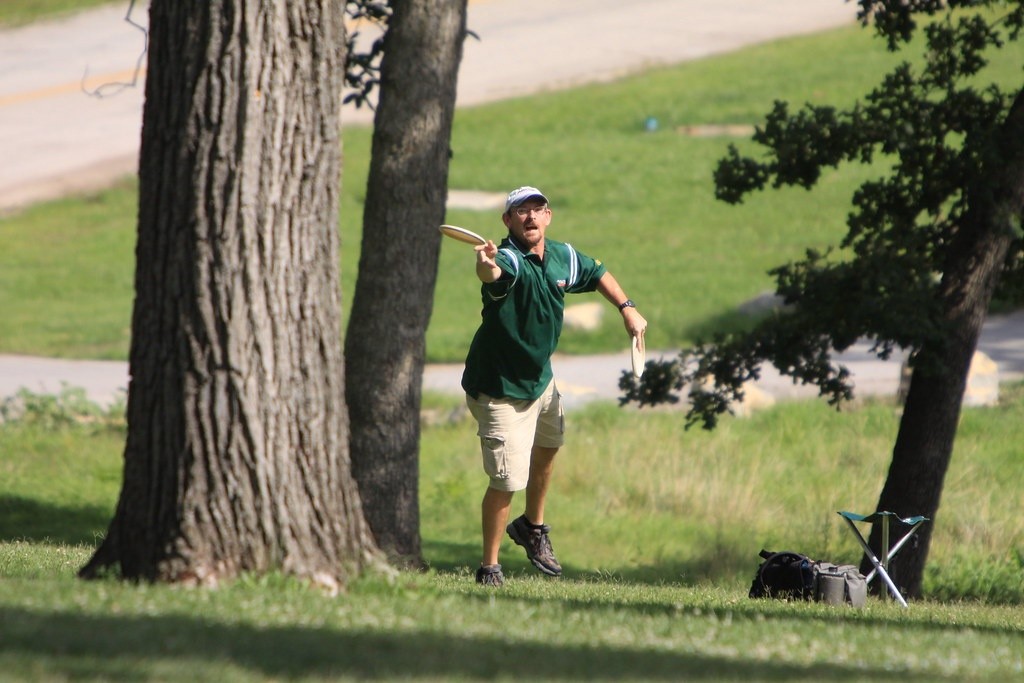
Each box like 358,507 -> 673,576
462,186 -> 648,589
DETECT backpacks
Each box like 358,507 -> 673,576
748,550 -> 821,603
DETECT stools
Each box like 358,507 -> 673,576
834,509 -> 930,610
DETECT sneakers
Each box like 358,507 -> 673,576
476,562 -> 503,586
506,514 -> 563,576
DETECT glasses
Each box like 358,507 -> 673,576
509,207 -> 547,216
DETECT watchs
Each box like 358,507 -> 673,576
618,300 -> 635,313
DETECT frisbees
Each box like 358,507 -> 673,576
631,336 -> 646,378
438,224 -> 486,245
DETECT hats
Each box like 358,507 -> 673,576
505,186 -> 549,212
